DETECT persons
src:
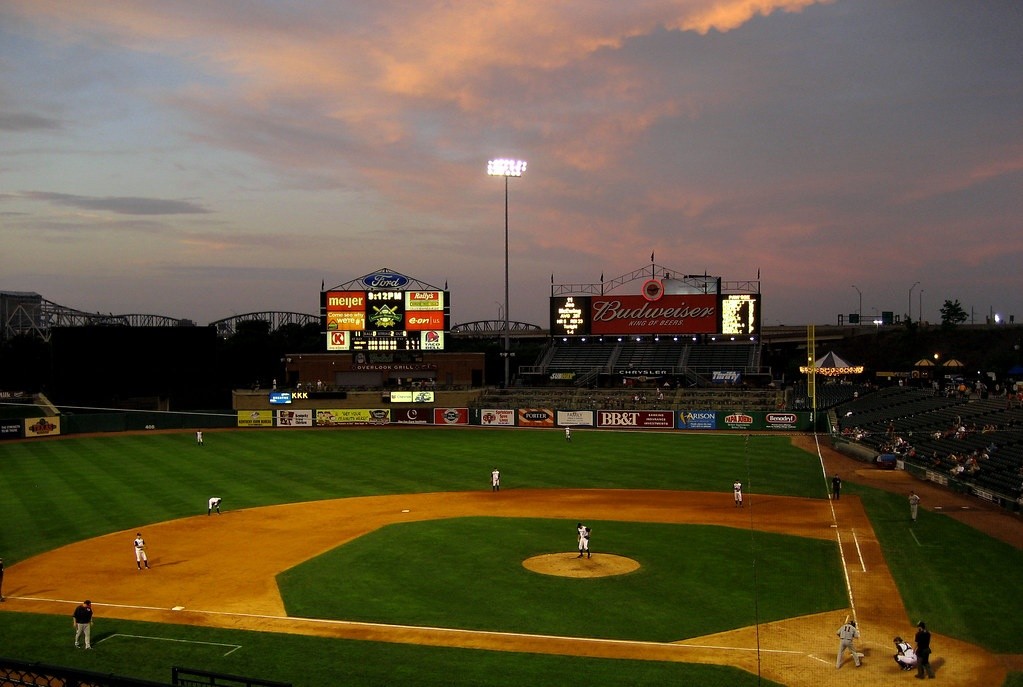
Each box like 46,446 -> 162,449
133,532 -> 150,570
584,378 -> 673,412
908,489 -> 920,522
893,637 -> 918,672
912,621 -> 935,679
250,376 -> 437,393
795,368 -> 1023,480
831,473 -> 841,500
563,425 -> 571,442
834,621 -> 862,669
490,467 -> 501,492
195,429 -> 205,447
208,497 -> 224,517
577,522 -> 592,560
73,599 -> 94,649
733,479 -> 743,508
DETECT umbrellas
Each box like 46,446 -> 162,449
912,358 -> 936,371
942,358 -> 964,367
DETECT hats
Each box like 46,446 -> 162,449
848,621 -> 855,627
86,600 -> 91,607
894,637 -> 903,641
917,621 -> 925,629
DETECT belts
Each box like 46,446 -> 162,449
841,638 -> 851,640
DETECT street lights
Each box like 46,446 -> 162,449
871,307 -> 879,321
852,285 -> 861,326
920,289 -> 927,323
909,282 -> 919,320
488,157 -> 526,388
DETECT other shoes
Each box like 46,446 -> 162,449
76,645 -> 80,648
145,567 -> 149,569
915,675 -> 924,679
588,554 -> 591,559
577,555 -> 583,558
137,567 -> 141,570
903,665 -> 914,671
87,647 -> 93,650
856,663 -> 862,667
